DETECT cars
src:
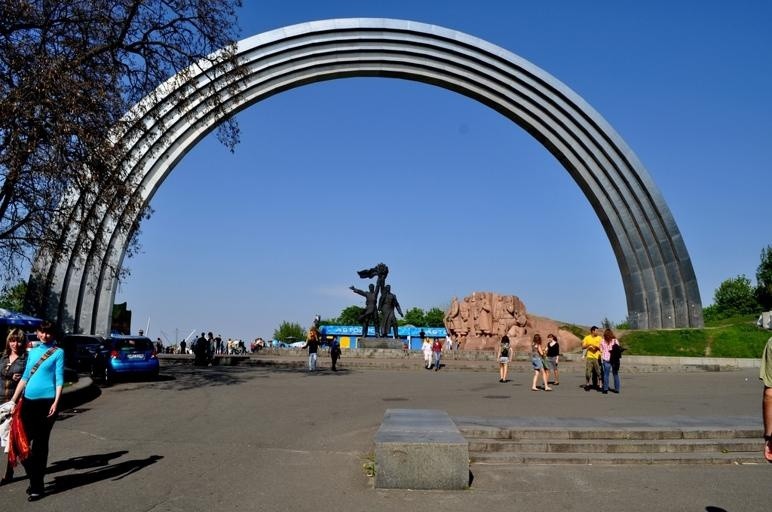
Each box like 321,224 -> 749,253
26,333 -> 58,346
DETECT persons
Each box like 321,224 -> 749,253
531,333 -> 559,391
371,262 -> 388,295
12,320 -> 65,501
496,336 -> 513,383
379,284 -> 405,339
302,330 -> 340,372
420,330 -> 459,371
191,332 -> 246,366
139,330 -> 143,336
759,337 -> 771,461
581,326 -> 620,393
348,284 -> 382,339
1,327 -> 29,484
250,338 -> 285,354
156,338 -> 187,354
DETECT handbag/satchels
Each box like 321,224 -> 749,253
8,398 -> 30,467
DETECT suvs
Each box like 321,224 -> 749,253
89,336 -> 162,385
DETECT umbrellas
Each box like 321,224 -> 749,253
2,312 -> 43,327
0,307 -> 18,321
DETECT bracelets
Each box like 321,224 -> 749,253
763,434 -> 771,441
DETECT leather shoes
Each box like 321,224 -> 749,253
26,486 -> 44,501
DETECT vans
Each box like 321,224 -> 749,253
59,334 -> 108,366
